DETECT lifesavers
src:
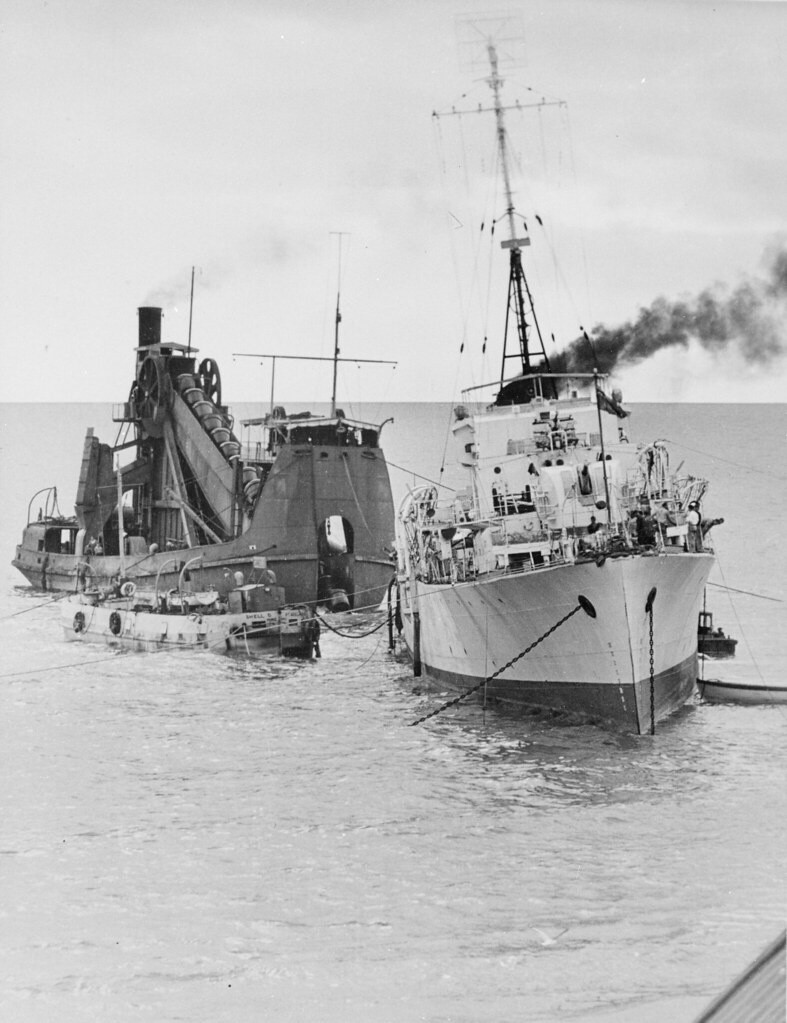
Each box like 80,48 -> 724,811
110,611 -> 121,634
73,611 -> 85,632
121,581 -> 136,597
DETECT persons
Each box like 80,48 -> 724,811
636,510 -> 656,544
587,515 -> 601,534
685,505 -> 701,553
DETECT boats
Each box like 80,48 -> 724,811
696,677 -> 787,704
131,590 -> 219,612
8,266 -> 400,616
56,561 -> 322,662
696,611 -> 738,653
78,581 -> 118,604
391,12 -> 715,738
